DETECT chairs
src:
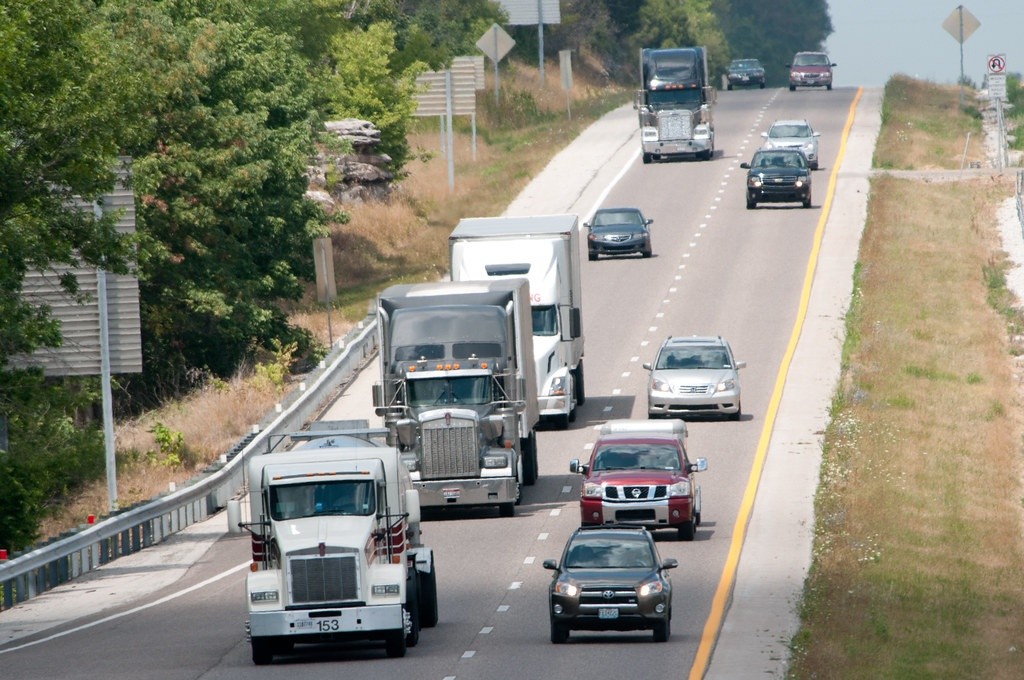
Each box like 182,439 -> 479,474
571,544 -> 598,567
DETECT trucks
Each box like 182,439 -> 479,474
632,46 -> 717,163
226,418 -> 440,665
446,215 -> 585,431
367,282 -> 541,518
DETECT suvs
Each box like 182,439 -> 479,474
543,523 -> 678,643
569,416 -> 709,542
760,117 -> 822,170
739,148 -> 819,211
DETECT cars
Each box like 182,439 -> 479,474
784,50 -> 838,91
724,57 -> 767,91
583,207 -> 654,261
641,334 -> 747,421
